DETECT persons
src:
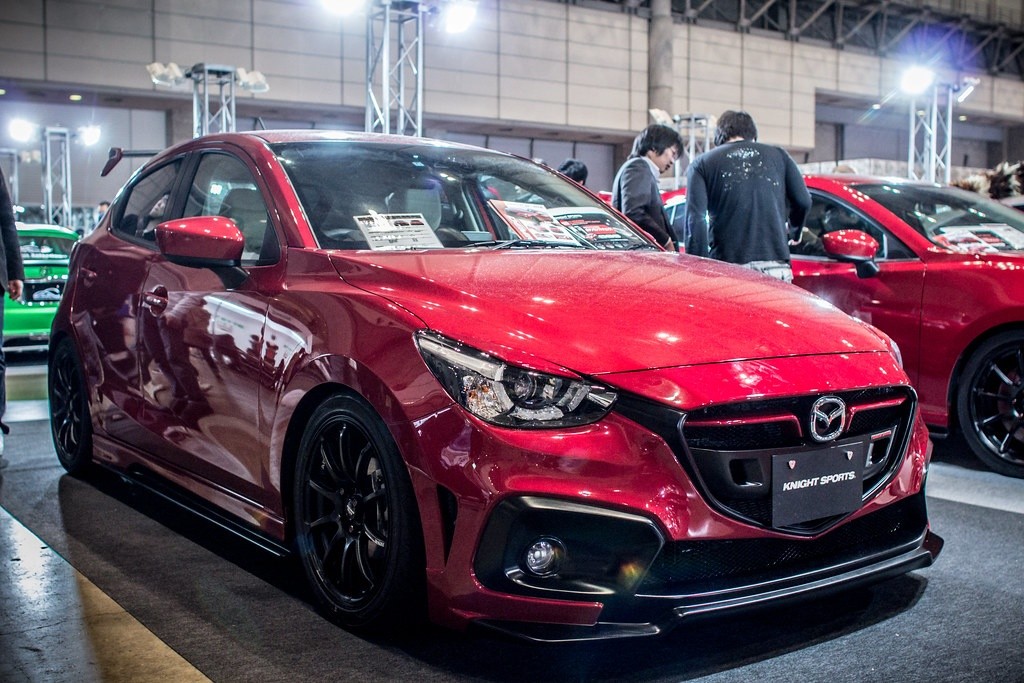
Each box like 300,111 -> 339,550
0,166 -> 26,436
102,287 -> 278,428
801,200 -> 868,260
513,156 -> 558,209
684,110 -> 813,285
611,123 -> 685,254
99,201 -> 111,221
557,158 -> 588,187
121,190 -> 173,242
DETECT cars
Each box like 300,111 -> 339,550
49,129 -> 945,650
897,197 -> 1024,268
2,224 -> 79,361
663,174 -> 1024,479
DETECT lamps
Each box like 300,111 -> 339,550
954,75 -> 981,103
649,108 -> 673,127
146,60 -> 185,90
236,67 -> 268,93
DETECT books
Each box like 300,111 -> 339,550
487,198 -> 585,250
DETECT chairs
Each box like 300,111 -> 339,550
219,189 -> 268,254
384,178 -> 461,245
